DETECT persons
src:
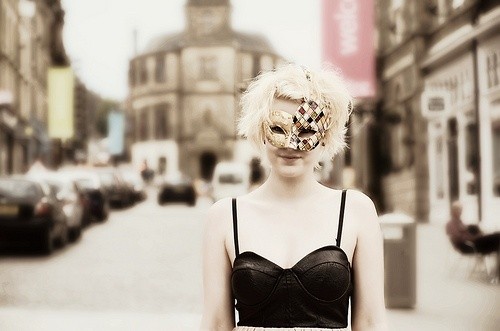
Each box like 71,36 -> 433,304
446,201 -> 500,256
200,64 -> 386,331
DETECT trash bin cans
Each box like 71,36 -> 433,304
379,212 -> 416,308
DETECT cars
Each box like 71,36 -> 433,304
157,171 -> 198,207
0,166 -> 148,254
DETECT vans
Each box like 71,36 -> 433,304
210,160 -> 271,202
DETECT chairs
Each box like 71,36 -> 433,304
450,235 -> 492,286
